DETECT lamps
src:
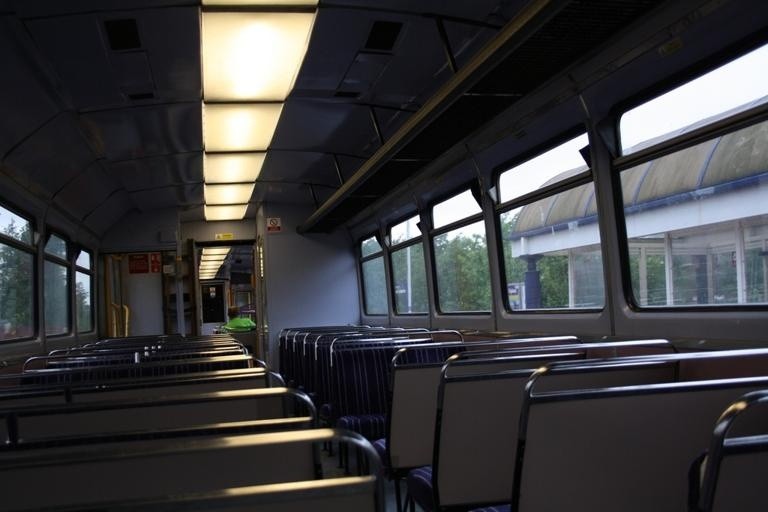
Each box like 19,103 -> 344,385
194,2 -> 320,222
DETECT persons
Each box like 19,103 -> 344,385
222,306 -> 256,332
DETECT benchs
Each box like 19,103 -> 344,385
0,326 -> 768,511
